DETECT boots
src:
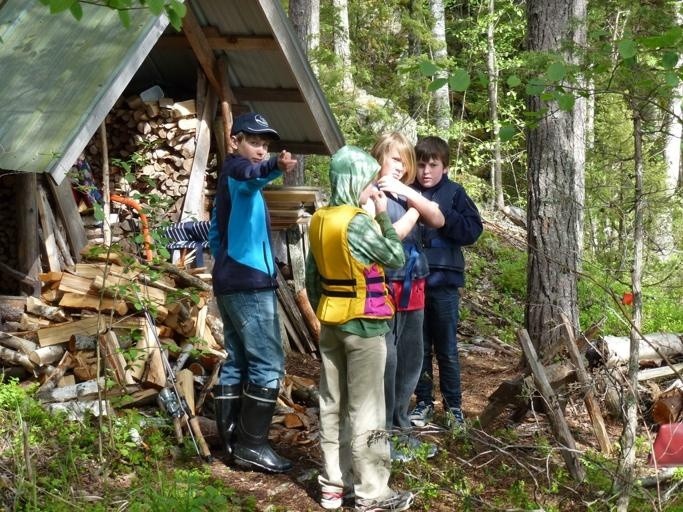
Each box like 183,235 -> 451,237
213,382 -> 241,461
231,380 -> 294,473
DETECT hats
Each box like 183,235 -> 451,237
230,113 -> 280,140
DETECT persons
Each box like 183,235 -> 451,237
304,144 -> 416,512
363,130 -> 439,462
409,134 -> 484,435
209,110 -> 298,474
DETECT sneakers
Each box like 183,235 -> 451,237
320,485 -> 355,510
443,407 -> 463,426
355,491 -> 414,512
390,430 -> 438,462
409,400 -> 433,426
